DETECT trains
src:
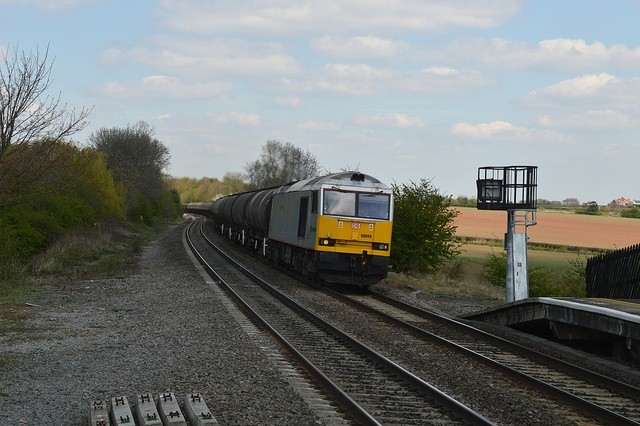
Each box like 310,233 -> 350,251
181,170 -> 394,288
